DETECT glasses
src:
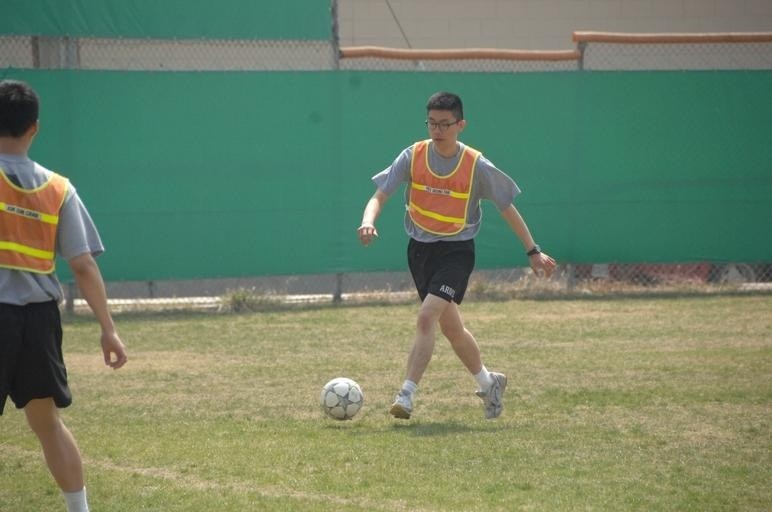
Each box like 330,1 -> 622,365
424,118 -> 460,132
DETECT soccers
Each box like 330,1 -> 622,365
320,377 -> 363,421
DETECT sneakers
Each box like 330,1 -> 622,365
389,389 -> 413,419
475,370 -> 508,420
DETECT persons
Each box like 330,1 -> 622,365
356,90 -> 555,421
1,80 -> 125,512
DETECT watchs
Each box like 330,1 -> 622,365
527,244 -> 542,257
